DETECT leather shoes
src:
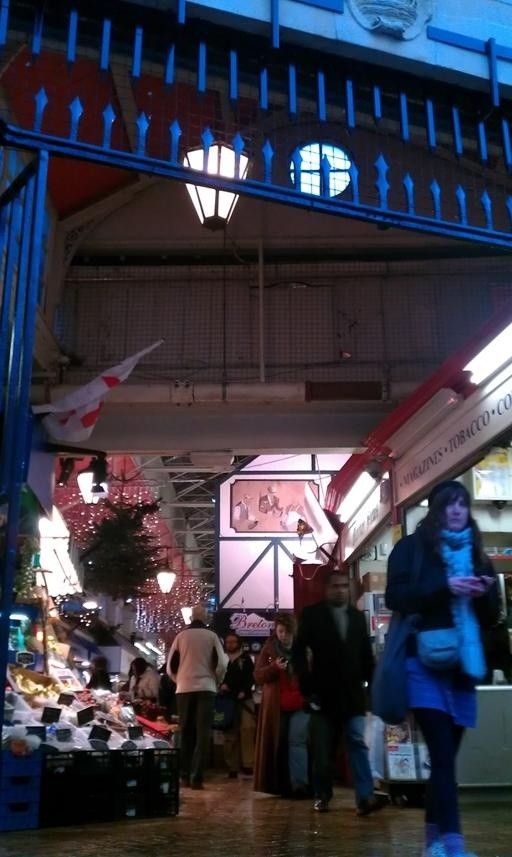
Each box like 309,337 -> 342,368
355,798 -> 389,814
312,795 -> 330,812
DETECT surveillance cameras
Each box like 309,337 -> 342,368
493,500 -> 506,510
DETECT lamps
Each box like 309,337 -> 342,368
54,457 -> 83,488
157,546 -> 181,594
182,143 -> 249,231
296,519 -> 342,565
88,452 -> 108,494
291,552 -> 333,581
382,387 -> 464,459
179,605 -> 192,626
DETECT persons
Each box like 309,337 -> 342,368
127,657 -> 160,708
120,660 -> 156,693
157,661 -> 177,708
382,476 -> 506,857
159,604 -> 232,792
232,490 -> 255,522
288,566 -> 391,821
84,655 -> 112,692
248,611 -> 314,802
212,631 -> 254,782
257,483 -> 282,518
283,497 -> 307,523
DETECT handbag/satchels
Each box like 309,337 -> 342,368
418,627 -> 462,669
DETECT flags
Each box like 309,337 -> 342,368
24,336 -> 156,446
